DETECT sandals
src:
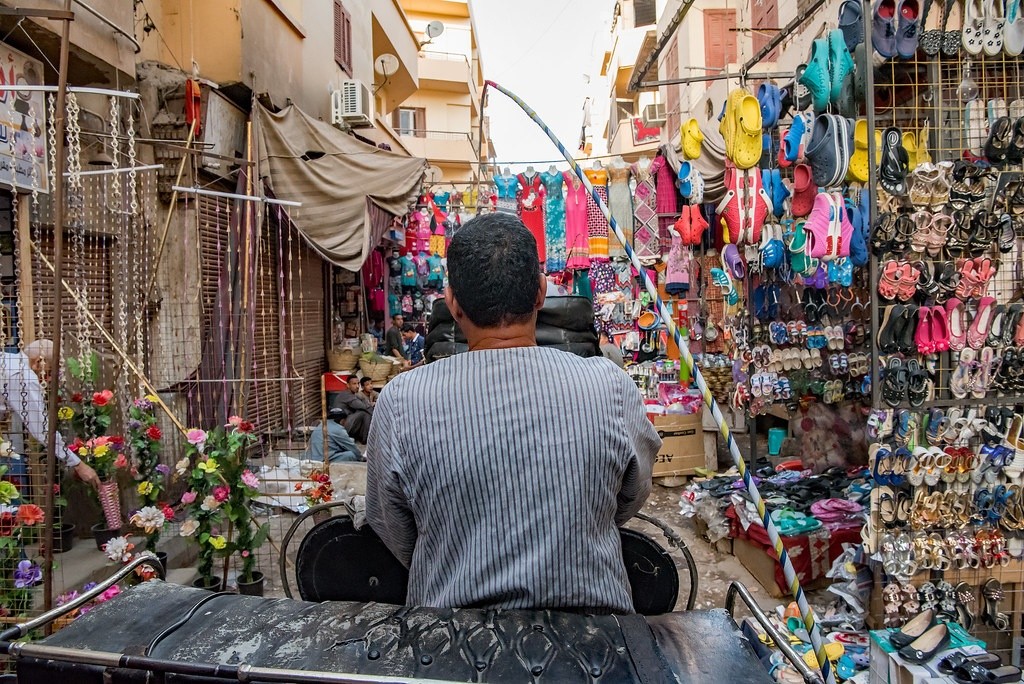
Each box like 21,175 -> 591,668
708,318 -> 871,419
869,98 -> 1024,305
862,483 -> 1024,580
881,578 -> 1010,634
867,347 -> 1024,486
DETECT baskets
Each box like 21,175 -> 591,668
358,355 -> 394,381
326,345 -> 359,371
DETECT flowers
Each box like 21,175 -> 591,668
0,350 -> 334,630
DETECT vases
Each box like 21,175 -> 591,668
145,551 -> 167,579
51,521 -> 76,554
191,575 -> 221,593
91,521 -> 124,551
235,570 -> 265,597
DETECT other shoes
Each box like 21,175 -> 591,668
889,607 -> 938,650
877,297 -> 1024,357
897,623 -> 951,665
871,0 -> 1024,59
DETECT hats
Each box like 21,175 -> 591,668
327,407 -> 348,417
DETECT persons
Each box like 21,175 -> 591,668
0,339 -> 100,617
494,150 -> 689,294
361,376 -> 380,406
367,313 -> 426,370
365,213 -> 664,616
310,408 -> 367,464
596,330 -> 623,368
333,375 -> 374,444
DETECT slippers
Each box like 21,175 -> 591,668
953,660 -> 1022,684
691,204 -> 709,245
677,161 -> 694,199
936,651 -> 1001,675
710,0 -> 932,322
679,118 -> 704,160
689,169 -> 704,205
673,205 -> 692,246
685,452 -> 875,537
753,600 -> 871,684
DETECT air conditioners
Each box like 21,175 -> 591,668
643,103 -> 666,127
341,80 -> 378,130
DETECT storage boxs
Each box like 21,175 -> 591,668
868,621 -> 1024,684
638,397 -> 869,598
322,367 -> 358,392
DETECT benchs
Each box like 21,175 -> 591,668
424,293 -> 605,364
0,550 -> 827,684
